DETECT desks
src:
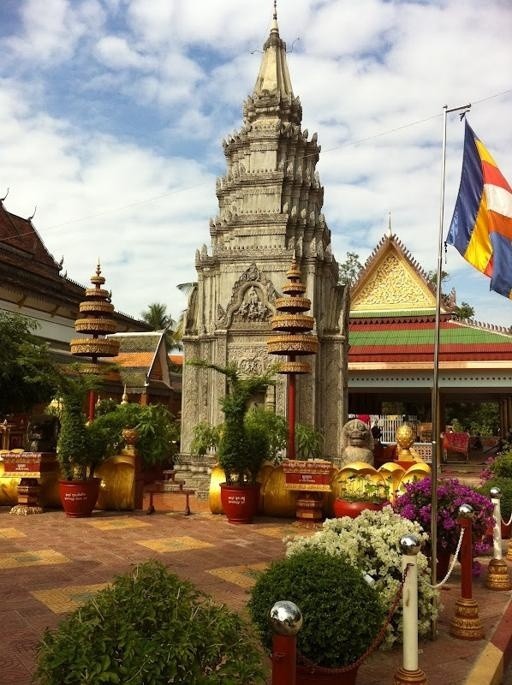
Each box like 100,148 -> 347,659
441,432 -> 470,464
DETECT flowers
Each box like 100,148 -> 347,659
281,504 -> 446,654
388,475 -> 499,578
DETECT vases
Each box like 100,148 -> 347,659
421,542 -> 450,580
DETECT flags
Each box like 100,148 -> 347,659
445,116 -> 512,300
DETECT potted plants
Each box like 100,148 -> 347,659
332,473 -> 394,521
241,542 -> 387,684
17,339 -> 181,518
475,476 -> 512,539
186,355 -> 326,525
450,418 -> 466,433
